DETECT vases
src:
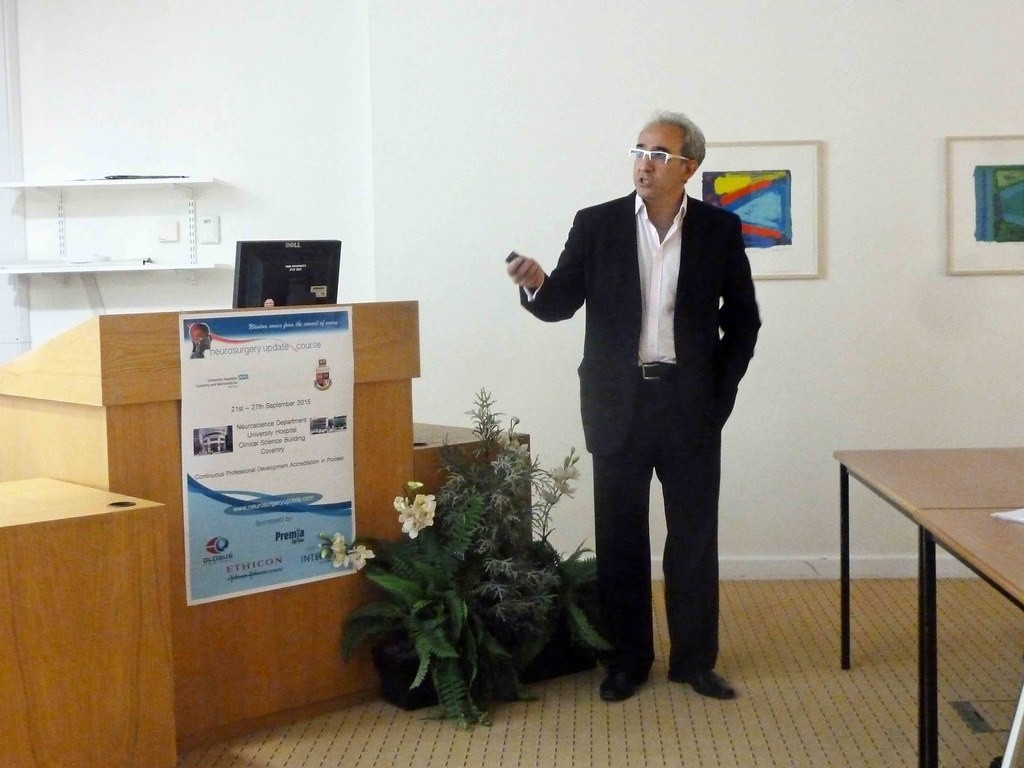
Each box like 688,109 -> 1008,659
374,631 -> 439,711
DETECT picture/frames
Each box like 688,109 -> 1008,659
685,140 -> 827,280
944,135 -> 1024,276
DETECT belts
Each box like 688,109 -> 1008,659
638,363 -> 674,381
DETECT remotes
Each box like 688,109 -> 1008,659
504,251 -> 526,266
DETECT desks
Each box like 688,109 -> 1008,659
411,423 -> 532,545
833,446 -> 1024,768
914,507 -> 1024,768
0,479 -> 178,768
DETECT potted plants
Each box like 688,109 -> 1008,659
443,390 -> 611,678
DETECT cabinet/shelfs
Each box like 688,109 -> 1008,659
0,177 -> 215,275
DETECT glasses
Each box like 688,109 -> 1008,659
630,148 -> 690,165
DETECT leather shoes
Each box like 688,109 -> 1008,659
669,662 -> 734,699
600,664 -> 649,700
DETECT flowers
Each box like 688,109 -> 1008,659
312,481 -> 537,731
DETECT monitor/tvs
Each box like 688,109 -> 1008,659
233,240 -> 342,309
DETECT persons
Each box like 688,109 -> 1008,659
504,112 -> 763,701
